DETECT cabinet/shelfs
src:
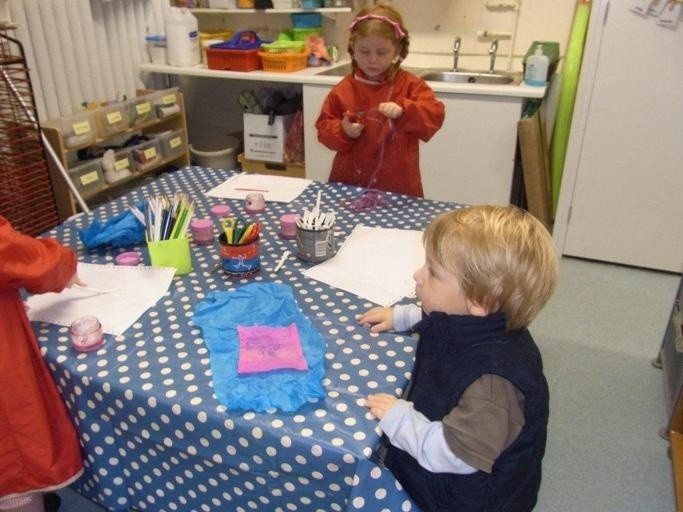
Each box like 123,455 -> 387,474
42,88 -> 190,224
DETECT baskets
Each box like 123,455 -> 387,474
257,38 -> 308,72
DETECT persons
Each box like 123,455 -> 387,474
315,4 -> 445,198
357,202 -> 559,512
1,215 -> 86,512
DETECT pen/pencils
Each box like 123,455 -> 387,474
235,188 -> 270,192
274,250 -> 292,273
295,191 -> 335,230
129,192 -> 195,241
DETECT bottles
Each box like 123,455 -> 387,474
245,193 -> 265,214
280,214 -> 301,240
69,316 -> 104,352
191,220 -> 215,245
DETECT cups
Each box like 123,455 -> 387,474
297,217 -> 335,263
145,228 -> 193,276
218,229 -> 261,279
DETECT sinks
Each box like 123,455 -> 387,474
419,70 -> 514,85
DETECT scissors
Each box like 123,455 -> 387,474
343,111 -> 365,125
222,217 -> 260,246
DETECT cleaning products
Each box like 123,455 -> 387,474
524,44 -> 549,86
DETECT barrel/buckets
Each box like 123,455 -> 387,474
163,6 -> 202,66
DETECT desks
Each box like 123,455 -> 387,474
18,166 -> 476,512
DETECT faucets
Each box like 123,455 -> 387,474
487,38 -> 498,73
450,36 -> 462,72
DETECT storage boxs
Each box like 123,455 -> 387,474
243,113 -> 299,164
57,87 -> 185,194
237,153 -> 305,179
204,47 -> 263,71
